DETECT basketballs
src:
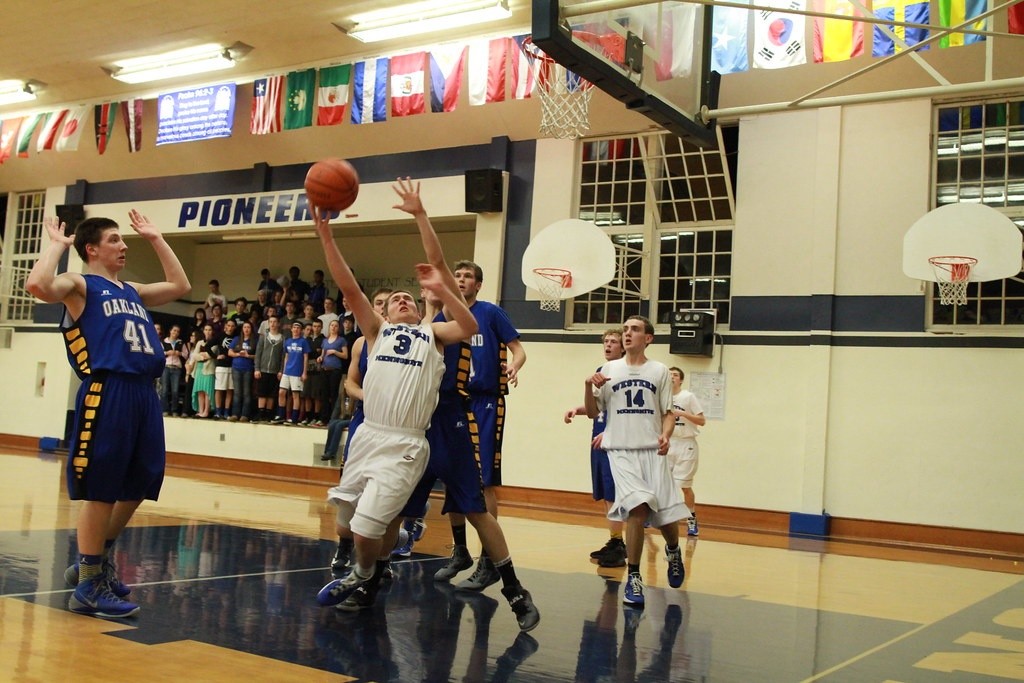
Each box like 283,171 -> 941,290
304,158 -> 359,211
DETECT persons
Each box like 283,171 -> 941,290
668,367 -> 706,535
564,329 -> 627,567
585,317 -> 685,608
23,209 -> 192,619
303,176 -> 540,633
110,514 -> 682,683
153,266 -> 425,430
320,378 -> 358,461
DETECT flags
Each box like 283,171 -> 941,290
0,1 -> 1024,167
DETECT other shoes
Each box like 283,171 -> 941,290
321,453 -> 335,461
162,410 -> 329,428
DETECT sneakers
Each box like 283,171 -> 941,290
382,563 -> 393,579
597,540 -> 626,567
591,539 -> 627,559
456,556 -> 500,592
331,538 -> 355,569
68,572 -> 140,617
687,516 -> 699,536
317,571 -> 362,606
623,572 -> 644,604
346,576 -> 377,604
394,528 -> 409,550
665,543 -> 684,588
398,539 -> 415,556
64,563 -> 132,599
435,544 -> 473,581
502,586 -> 540,631
412,501 -> 431,541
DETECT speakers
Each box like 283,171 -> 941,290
58,208 -> 76,237
464,168 -> 503,213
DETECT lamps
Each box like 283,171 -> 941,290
332,0 -> 514,43
579,210 -> 625,225
0,79 -> 46,107
100,41 -> 239,86
